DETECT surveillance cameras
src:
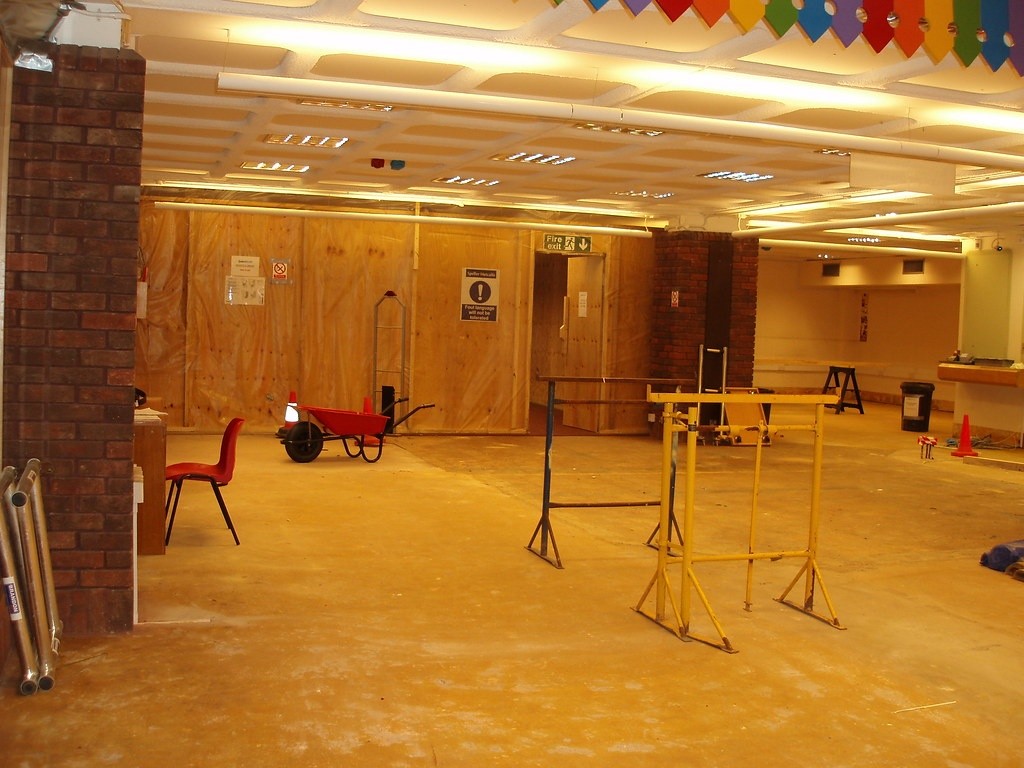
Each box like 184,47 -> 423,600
993,245 -> 1002,251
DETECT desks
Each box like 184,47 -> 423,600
133,415 -> 167,556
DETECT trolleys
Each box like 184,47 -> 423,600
279,397 -> 437,464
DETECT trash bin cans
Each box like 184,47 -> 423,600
900,382 -> 935,432
757,387 -> 775,424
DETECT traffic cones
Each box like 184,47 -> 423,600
356,397 -> 387,445
949,413 -> 980,458
276,392 -> 300,437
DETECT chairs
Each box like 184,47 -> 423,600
165,417 -> 244,547
